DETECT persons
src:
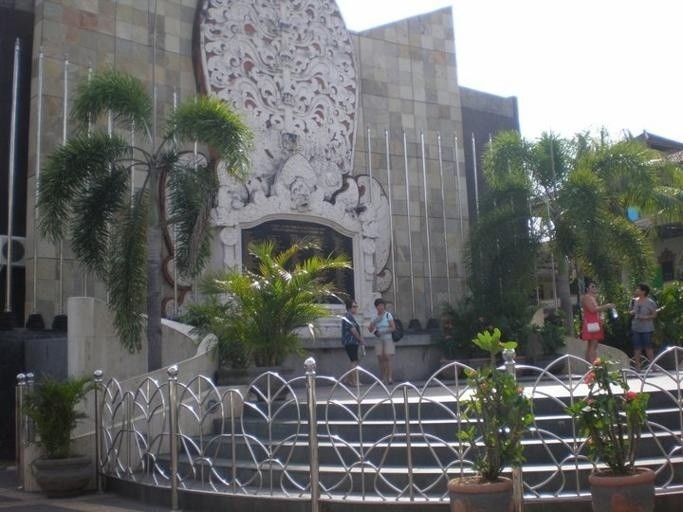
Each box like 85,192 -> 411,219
366,297 -> 403,385
622,283 -> 662,373
339,299 -> 366,386
578,277 -> 617,367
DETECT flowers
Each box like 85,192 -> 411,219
564,354 -> 655,476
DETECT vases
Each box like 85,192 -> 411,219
588,466 -> 655,512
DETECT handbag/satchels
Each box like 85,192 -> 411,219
357,343 -> 366,358
629,297 -> 636,327
587,322 -> 601,332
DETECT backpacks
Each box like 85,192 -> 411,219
386,312 -> 404,342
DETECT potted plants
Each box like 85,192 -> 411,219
447,328 -> 537,512
16,368 -> 106,498
177,231 -> 355,401
421,295 -> 570,380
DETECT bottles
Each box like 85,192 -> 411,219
611,308 -> 618,318
